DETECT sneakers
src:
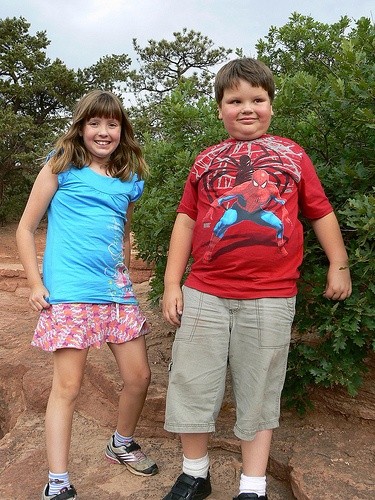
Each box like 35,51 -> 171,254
162,469 -> 211,500
41,483 -> 77,500
104,434 -> 158,476
231,492 -> 267,500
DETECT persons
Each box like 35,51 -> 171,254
160,57 -> 352,500
17,89 -> 159,500
